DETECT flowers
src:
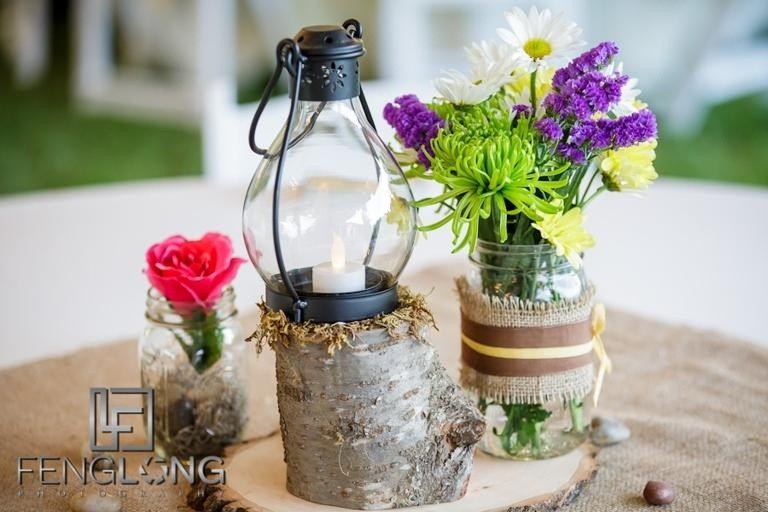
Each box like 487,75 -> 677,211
140,232 -> 251,377
381,6 -> 661,450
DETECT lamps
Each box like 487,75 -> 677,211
241,19 -> 418,324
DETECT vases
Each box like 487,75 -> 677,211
141,287 -> 251,459
456,232 -> 606,463
